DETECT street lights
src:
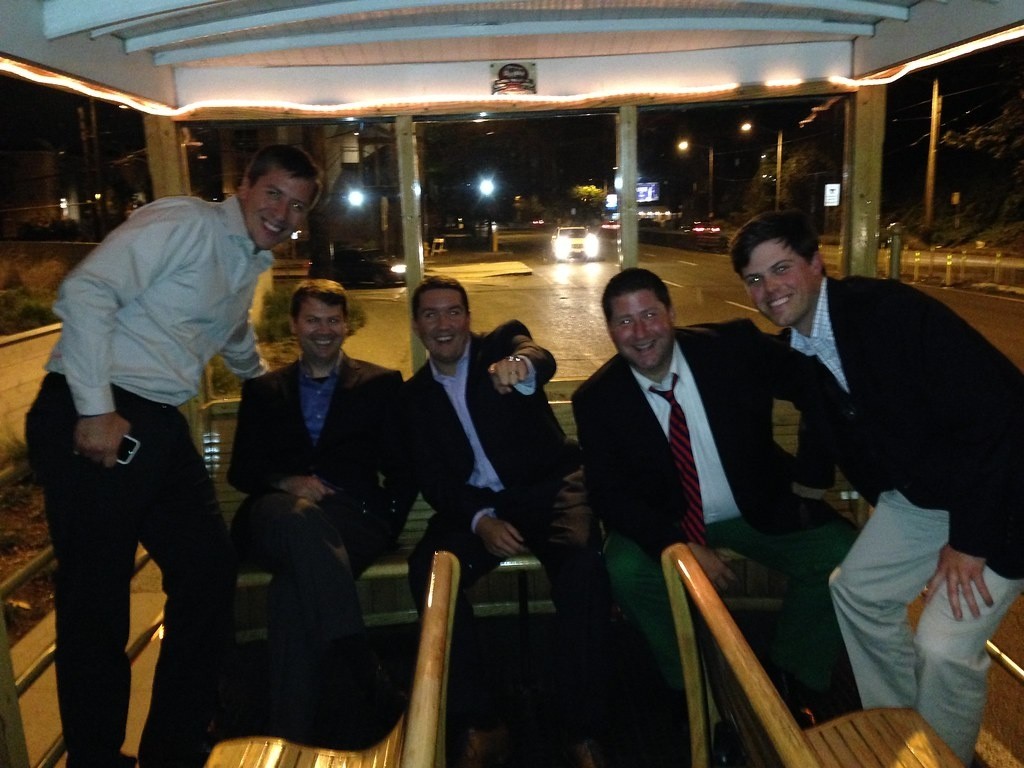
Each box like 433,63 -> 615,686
679,140 -> 715,220
740,122 -> 783,210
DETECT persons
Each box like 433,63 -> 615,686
404,274 -> 612,768
730,211 -> 1024,768
571,268 -> 857,768
23,142 -> 329,768
226,279 -> 420,744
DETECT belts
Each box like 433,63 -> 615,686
117,391 -> 170,423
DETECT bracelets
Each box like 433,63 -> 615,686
506,353 -> 520,362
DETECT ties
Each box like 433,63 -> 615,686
647,375 -> 707,546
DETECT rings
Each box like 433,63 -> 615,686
509,370 -> 519,374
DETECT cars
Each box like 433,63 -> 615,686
310,245 -> 408,290
551,226 -> 598,259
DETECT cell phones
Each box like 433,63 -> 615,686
116,434 -> 140,465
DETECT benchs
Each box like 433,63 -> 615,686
659,542 -> 965,768
199,378 -> 871,651
201,549 -> 458,768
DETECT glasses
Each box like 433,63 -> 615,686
248,187 -> 309,214
